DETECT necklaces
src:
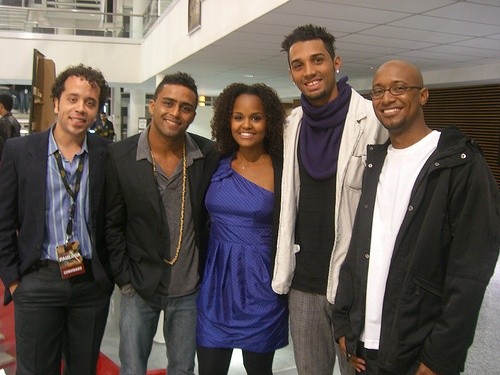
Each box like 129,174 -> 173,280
150,138 -> 187,265
242,156 -> 265,173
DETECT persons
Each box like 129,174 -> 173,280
271,25 -> 390,375
94,111 -> 115,141
195,82 -> 291,375
0,93 -> 21,155
105,71 -> 219,375
0,63 -> 115,375
333,60 -> 500,375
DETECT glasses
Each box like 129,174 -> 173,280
370,84 -> 422,99
